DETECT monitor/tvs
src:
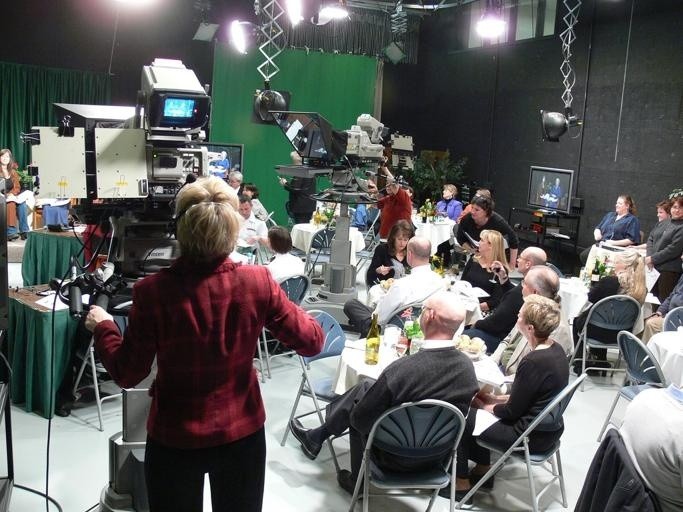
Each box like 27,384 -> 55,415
197,142 -> 243,183
528,165 -> 574,216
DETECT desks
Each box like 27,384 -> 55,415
6,223 -> 87,417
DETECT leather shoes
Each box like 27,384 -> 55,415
288,418 -> 322,460
337,469 -> 364,500
431,467 -> 494,505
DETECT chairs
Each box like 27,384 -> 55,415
71,295 -> 133,432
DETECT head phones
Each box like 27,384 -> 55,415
169,201 -> 200,238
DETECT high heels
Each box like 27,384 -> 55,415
573,362 -> 611,376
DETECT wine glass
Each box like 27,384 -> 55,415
384,324 -> 407,361
488,262 -> 500,284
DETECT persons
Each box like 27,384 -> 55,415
0,148 -> 31,242
183,150 -> 683,512
85,172 -> 326,511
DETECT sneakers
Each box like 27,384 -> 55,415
6,232 -> 28,241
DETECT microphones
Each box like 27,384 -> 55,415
167,174 -> 198,221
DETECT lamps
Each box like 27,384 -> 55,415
249,1 -> 292,128
538,0 -> 584,141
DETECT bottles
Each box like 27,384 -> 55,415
580,259 -> 604,288
314,206 -> 321,226
407,319 -> 421,354
364,311 -> 381,364
320,209 -> 328,226
411,204 -> 439,224
430,256 -> 441,271
